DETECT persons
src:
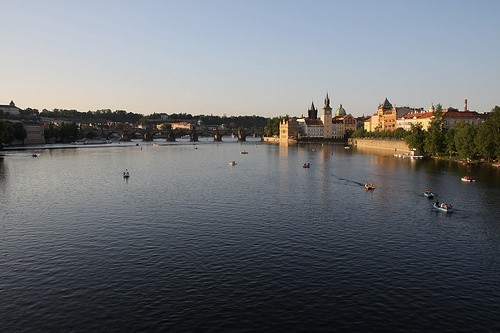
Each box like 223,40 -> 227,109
425,188 -> 432,194
435,200 -> 452,209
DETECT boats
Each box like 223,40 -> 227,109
460,178 -> 477,182
229,162 -> 236,166
302,165 -> 309,168
433,203 -> 453,212
241,152 -> 248,154
365,183 -> 375,190
394,153 -> 424,159
123,172 -> 129,177
424,191 -> 438,197
